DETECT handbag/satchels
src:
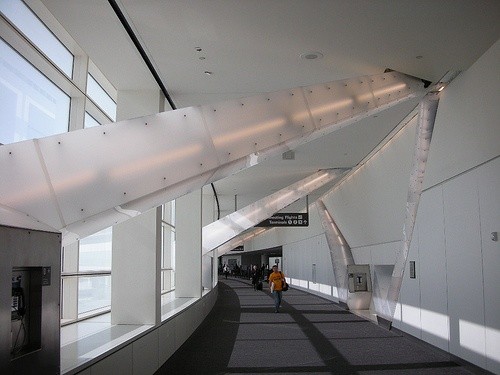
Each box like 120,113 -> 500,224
257,282 -> 263,290
282,281 -> 289,291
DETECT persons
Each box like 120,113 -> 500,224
269,265 -> 287,313
222,263 -> 274,284
252,265 -> 260,288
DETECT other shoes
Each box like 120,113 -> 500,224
279,305 -> 281,308
275,309 -> 278,312
